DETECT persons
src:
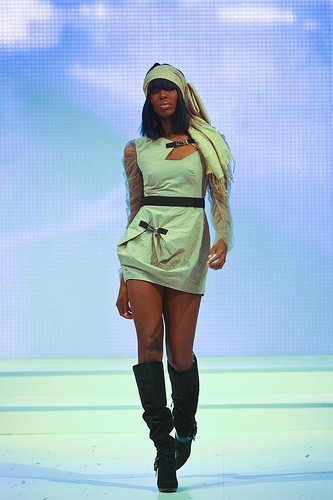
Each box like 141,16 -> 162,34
116,62 -> 236,492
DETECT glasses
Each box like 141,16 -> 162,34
147,82 -> 176,94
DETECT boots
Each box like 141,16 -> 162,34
167,350 -> 200,470
132,361 -> 178,494
